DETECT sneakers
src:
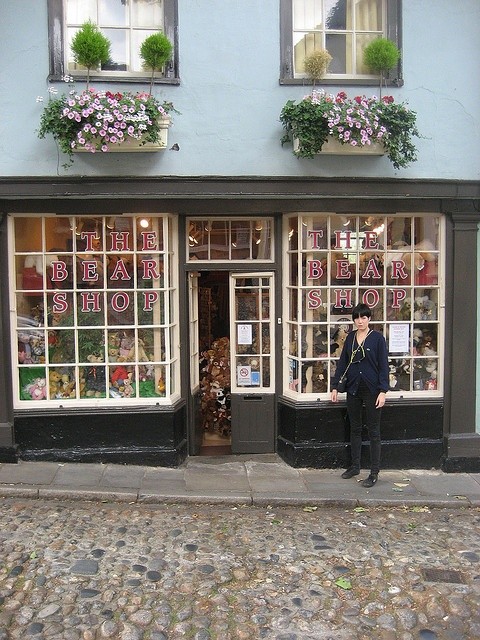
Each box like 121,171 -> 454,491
363,475 -> 378,487
342,467 -> 360,479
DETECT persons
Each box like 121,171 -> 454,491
331,304 -> 389,488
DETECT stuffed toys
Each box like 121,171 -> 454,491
18,329 -> 167,400
198,287 -> 218,356
81,350 -> 106,398
289,324 -> 438,358
24,238 -> 164,289
27,302 -> 60,327
318,238 -> 435,285
235,297 -> 270,388
198,322 -> 231,439
289,288 -> 437,321
289,358 -> 438,394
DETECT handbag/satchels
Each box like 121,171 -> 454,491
338,330 -> 373,393
